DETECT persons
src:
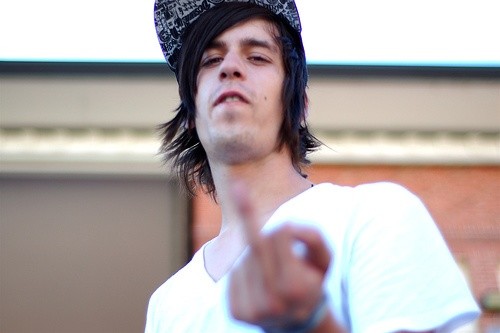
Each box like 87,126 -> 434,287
143,0 -> 482,333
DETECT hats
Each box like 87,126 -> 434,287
153,0 -> 302,71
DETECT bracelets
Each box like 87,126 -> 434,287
305,295 -> 332,333
